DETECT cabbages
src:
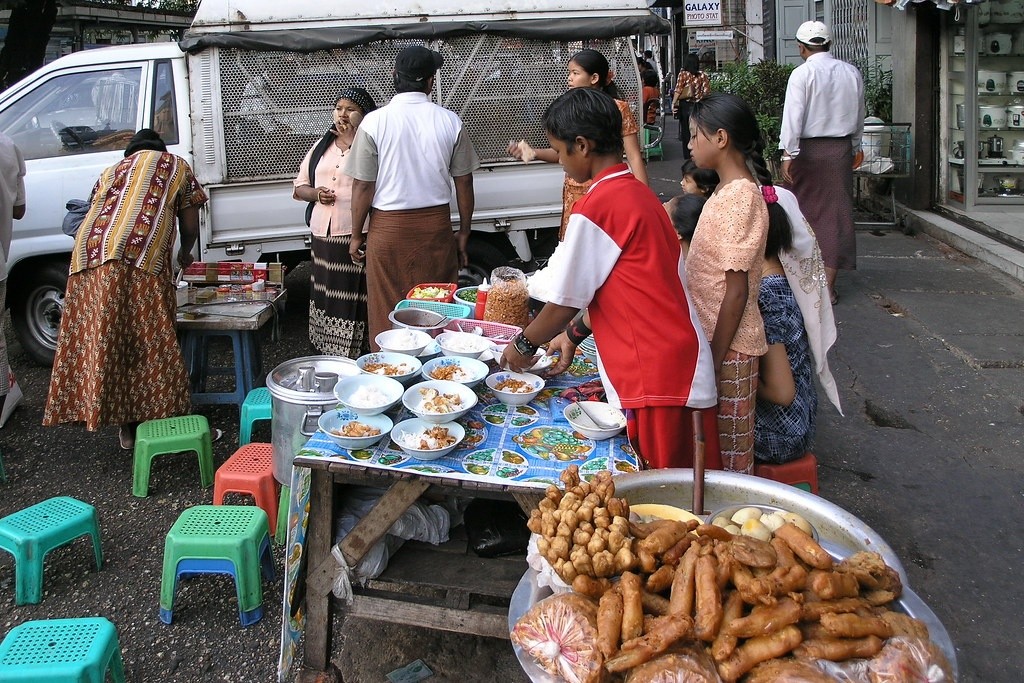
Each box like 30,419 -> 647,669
410,286 -> 451,299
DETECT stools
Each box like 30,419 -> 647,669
180,330 -> 264,423
642,125 -> 664,162
0,617 -> 125,683
159,506 -> 276,612
159,532 -> 276,627
240,387 -> 273,447
275,485 -> 290,545
665,98 -> 672,111
132,415 -> 215,497
0,496 -> 104,605
213,443 -> 282,536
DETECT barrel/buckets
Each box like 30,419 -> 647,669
267,356 -> 360,487
862,125 -> 885,165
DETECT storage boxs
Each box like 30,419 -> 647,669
183,262 -> 282,282
196,292 -> 217,302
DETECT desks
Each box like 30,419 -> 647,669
176,288 -> 288,331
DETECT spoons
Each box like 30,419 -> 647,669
456,323 -> 504,339
576,402 -> 620,429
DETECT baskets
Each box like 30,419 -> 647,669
395,300 -> 471,319
443,318 -> 523,344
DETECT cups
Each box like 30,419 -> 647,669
296,367 -> 315,391
313,372 -> 339,393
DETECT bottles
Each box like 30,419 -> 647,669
475,277 -> 491,321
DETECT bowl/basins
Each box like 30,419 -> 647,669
629,503 -> 704,538
489,344 -> 546,371
334,375 -> 405,416
507,469 -> 958,683
704,503 -> 819,544
390,418 -> 465,460
402,380 -> 478,424
563,401 -> 627,440
388,307 -> 450,340
356,352 -> 422,382
318,407 -> 394,450
577,333 -> 597,365
375,329 -> 431,357
435,332 -> 491,359
485,372 -> 545,407
422,356 -> 489,388
417,339 -> 441,357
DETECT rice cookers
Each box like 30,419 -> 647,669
953,0 -> 1024,195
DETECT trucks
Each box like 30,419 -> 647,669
0,1 -> 672,368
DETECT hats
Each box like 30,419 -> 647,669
123,128 -> 169,158
333,88 -> 377,114
393,46 -> 445,83
796,20 -> 831,45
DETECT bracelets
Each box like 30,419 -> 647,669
672,102 -> 676,104
348,145 -> 351,149
566,316 -> 592,346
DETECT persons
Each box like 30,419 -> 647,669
0,131 -> 26,419
347,47 -> 481,355
753,174 -> 845,466
91,70 -> 139,131
500,87 -> 722,470
672,53 -> 710,161
776,19 -> 865,304
635,48 -> 659,125
293,88 -> 377,360
40,128 -> 221,449
505,50 -> 650,239
682,93 -> 768,476
660,158 -> 721,261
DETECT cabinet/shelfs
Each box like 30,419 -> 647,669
946,5 -> 1024,213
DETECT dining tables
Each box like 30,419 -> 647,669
279,312 -> 643,682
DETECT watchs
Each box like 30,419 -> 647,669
780,155 -> 792,161
513,332 -> 539,357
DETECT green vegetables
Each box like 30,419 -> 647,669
457,288 -> 477,303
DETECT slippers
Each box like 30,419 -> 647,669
118,427 -> 135,449
210,427 -> 222,444
830,289 -> 839,305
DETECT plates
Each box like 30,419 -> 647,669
502,354 -> 553,375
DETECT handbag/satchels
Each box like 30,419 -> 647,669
678,71 -> 694,100
